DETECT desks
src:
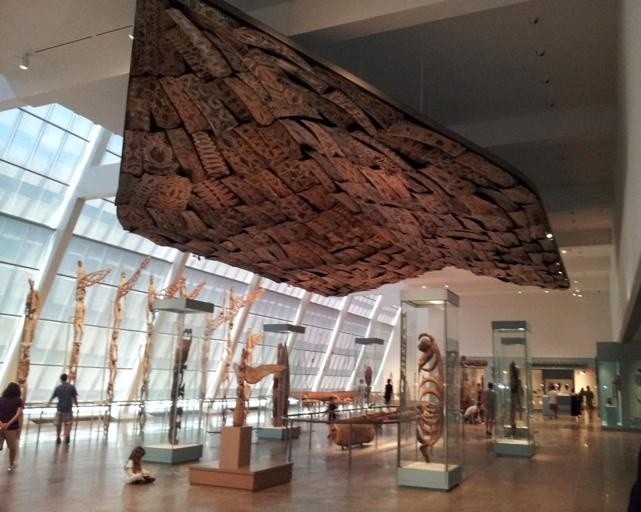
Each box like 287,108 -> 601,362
543,394 -> 571,416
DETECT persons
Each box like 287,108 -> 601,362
0,382 -> 25,472
322,378 -> 595,439
123,446 -> 155,484
46,373 -> 80,444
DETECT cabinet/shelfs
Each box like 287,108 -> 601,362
140,297 -> 214,465
491,321 -> 534,459
354,337 -> 384,393
396,288 -> 461,491
500,336 -> 531,438
255,324 -> 306,441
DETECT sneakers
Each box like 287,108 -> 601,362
141,474 -> 156,484
7,461 -> 18,472
55,433 -> 72,445
